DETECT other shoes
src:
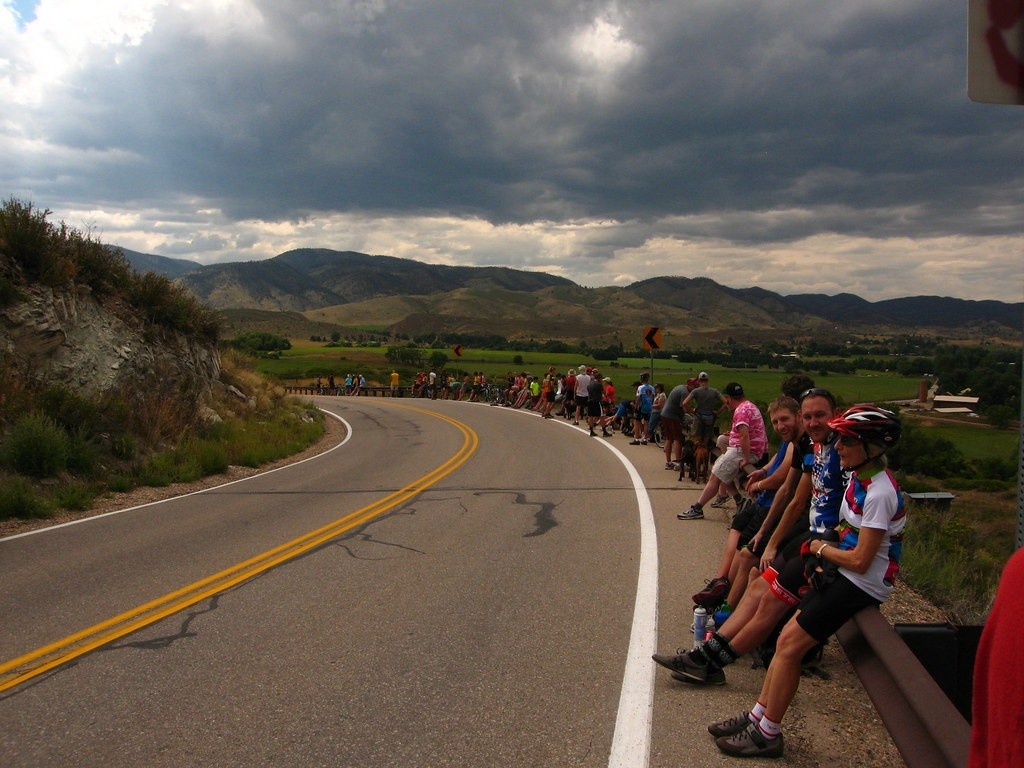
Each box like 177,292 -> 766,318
587,423 -> 680,471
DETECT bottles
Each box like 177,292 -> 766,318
706,616 -> 715,640
720,600 -> 730,613
694,605 -> 707,649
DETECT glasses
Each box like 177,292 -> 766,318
800,388 -> 828,399
837,434 -> 862,447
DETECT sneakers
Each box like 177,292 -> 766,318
693,601 -> 724,615
717,724 -> 784,758
711,494 -> 730,507
670,668 -> 726,685
652,648 -> 708,681
692,577 -> 729,605
708,711 -> 754,737
732,498 -> 752,517
677,506 -> 704,520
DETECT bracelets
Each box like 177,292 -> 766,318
761,468 -> 767,475
758,481 -> 763,490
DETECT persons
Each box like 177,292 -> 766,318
653,388 -> 847,685
709,406 -> 907,758
345,374 -> 365,396
390,370 -> 399,397
968,547 -> 1024,768
316,374 -> 336,396
411,364 -> 814,632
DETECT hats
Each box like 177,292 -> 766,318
578,365 -> 585,373
688,378 -> 699,386
586,367 -> 592,374
699,372 -> 708,379
641,372 -> 650,378
602,377 -> 612,383
568,369 -> 576,376
724,383 -> 743,395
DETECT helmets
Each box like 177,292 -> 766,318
826,405 -> 902,447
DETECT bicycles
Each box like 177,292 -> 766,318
654,412 -> 696,448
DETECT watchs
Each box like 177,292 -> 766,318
816,544 -> 827,558
